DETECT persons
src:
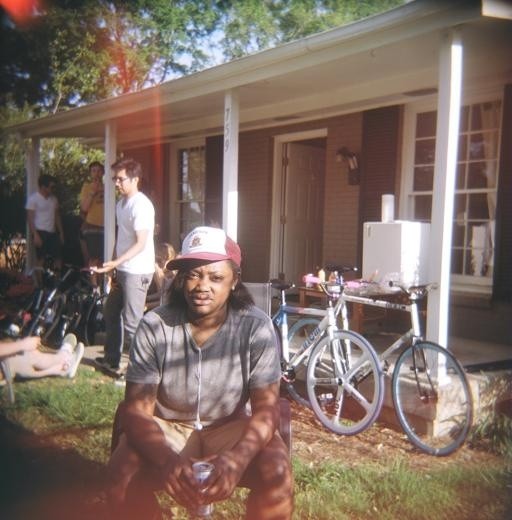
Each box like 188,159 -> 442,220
0,333 -> 84,385
90,158 -> 155,386
79,162 -> 104,258
112,227 -> 294,520
24,174 -> 65,268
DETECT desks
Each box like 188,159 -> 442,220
298,285 -> 427,350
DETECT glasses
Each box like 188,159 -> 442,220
112,176 -> 130,183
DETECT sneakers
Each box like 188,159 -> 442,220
94,358 -> 122,375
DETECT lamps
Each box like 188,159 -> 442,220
333,146 -> 360,184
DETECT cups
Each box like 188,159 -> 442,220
381,194 -> 394,224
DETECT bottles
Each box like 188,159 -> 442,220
318,269 -> 325,283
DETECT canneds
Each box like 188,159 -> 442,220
191,461 -> 214,516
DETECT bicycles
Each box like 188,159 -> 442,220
0,231 -> 110,348
269,269 -> 472,454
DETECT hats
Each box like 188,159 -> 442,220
166,226 -> 242,271
56,333 -> 84,378
